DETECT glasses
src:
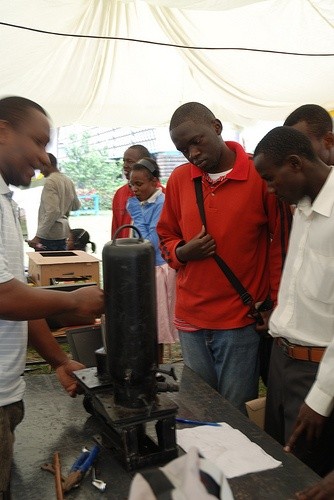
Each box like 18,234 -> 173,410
65,237 -> 73,242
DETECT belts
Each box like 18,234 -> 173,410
273,336 -> 324,362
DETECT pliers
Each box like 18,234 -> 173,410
63,447 -> 98,493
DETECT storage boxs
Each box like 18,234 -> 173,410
25,249 -> 103,288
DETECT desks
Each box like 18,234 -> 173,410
8,360 -> 321,500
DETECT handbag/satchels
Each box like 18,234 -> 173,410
253,297 -> 272,385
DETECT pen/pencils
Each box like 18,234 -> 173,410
175,417 -> 221,428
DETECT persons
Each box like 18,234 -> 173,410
0,97 -> 106,500
66,228 -> 95,254
28,152 -> 81,252
110,101 -> 334,500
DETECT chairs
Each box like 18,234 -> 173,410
66,326 -> 164,368
